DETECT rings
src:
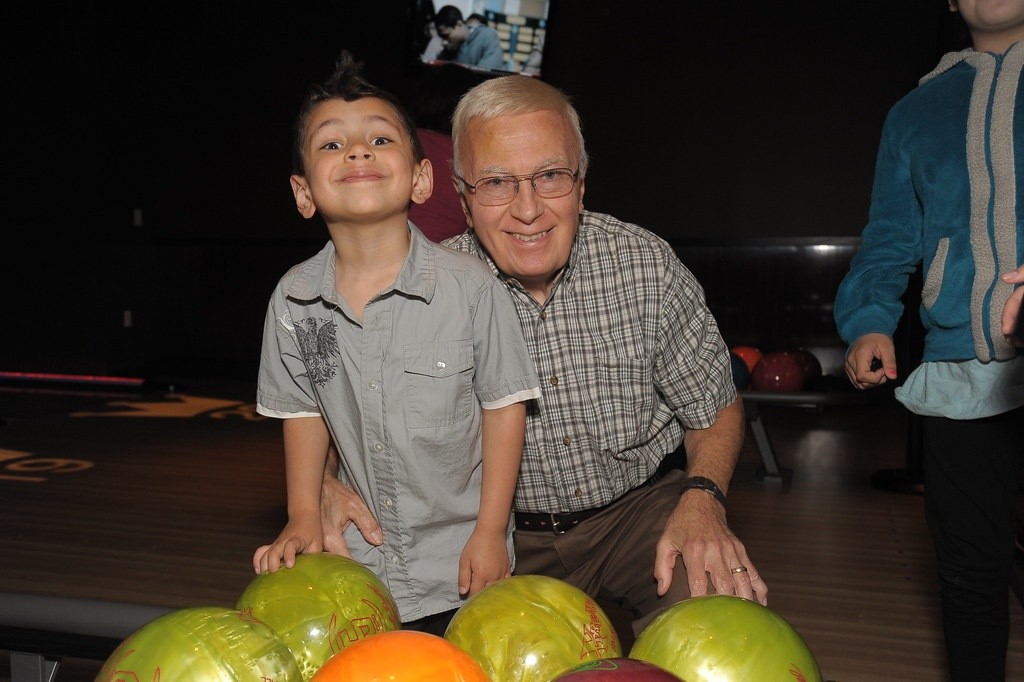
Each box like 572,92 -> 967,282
731,567 -> 747,573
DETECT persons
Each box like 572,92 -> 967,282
423,5 -> 504,68
832,0 -> 1024,681
320,76 -> 768,638
256,70 -> 541,636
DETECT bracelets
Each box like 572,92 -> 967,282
679,476 -> 727,510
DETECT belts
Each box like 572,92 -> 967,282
512,500 -> 621,535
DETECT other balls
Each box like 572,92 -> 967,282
90,552 -> 823,681
726,343 -> 846,396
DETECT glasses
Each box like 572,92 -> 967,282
455,162 -> 582,206
